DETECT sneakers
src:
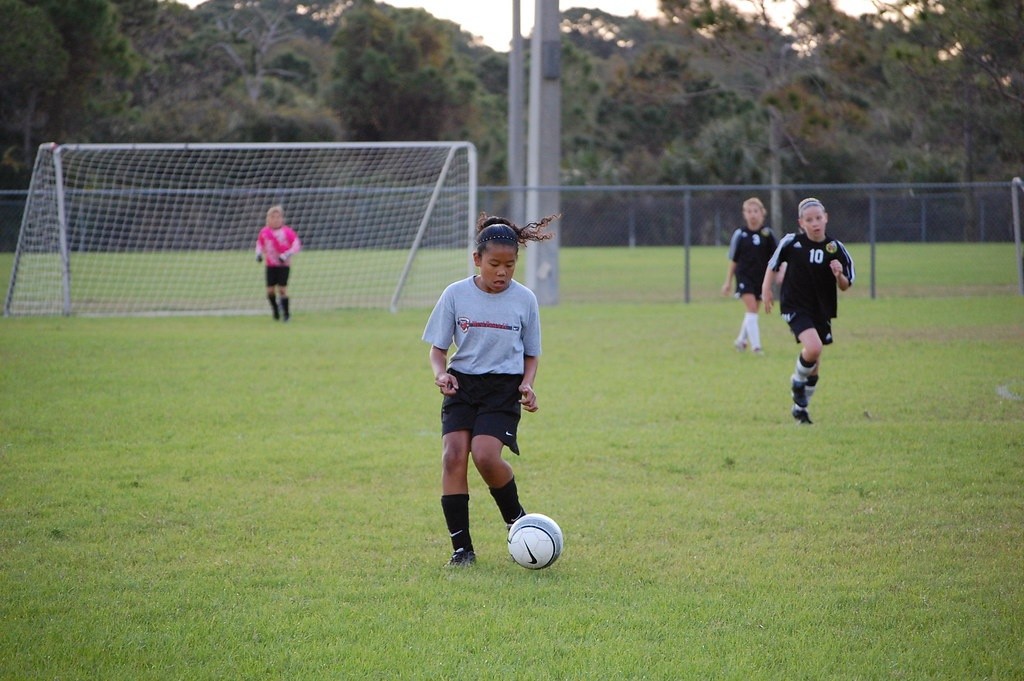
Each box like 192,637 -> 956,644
444,547 -> 476,568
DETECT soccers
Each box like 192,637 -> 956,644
505,511 -> 564,572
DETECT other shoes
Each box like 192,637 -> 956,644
274,306 -> 280,319
283,305 -> 289,320
792,409 -> 813,425
750,345 -> 763,355
791,378 -> 808,407
734,340 -> 747,352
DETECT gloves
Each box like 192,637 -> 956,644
278,257 -> 283,262
256,255 -> 262,262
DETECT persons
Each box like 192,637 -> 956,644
762,198 -> 856,423
255,207 -> 301,321
722,198 -> 788,352
422,214 -> 559,567
